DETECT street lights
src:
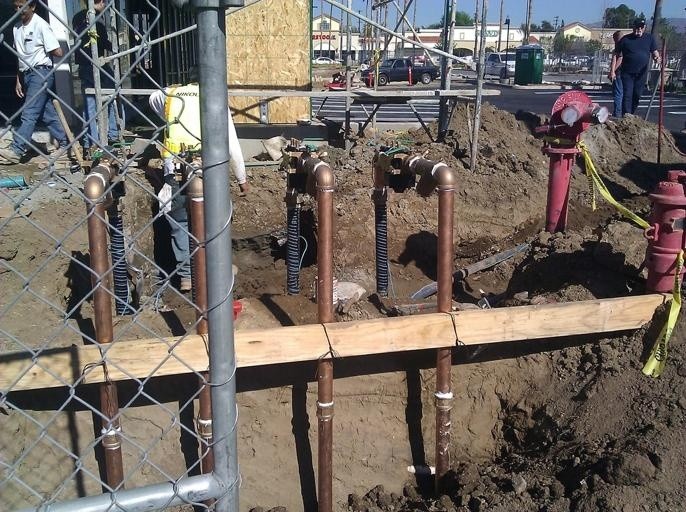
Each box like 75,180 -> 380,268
504,14 -> 511,81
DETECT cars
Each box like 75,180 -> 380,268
399,55 -> 480,65
540,53 -> 614,73
312,57 -> 342,65
651,53 -> 680,69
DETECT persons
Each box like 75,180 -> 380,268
149,62 -> 249,293
610,17 -> 662,115
71,0 -> 121,160
0,0 -> 75,164
607,30 -> 623,117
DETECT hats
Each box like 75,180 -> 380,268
633,18 -> 645,25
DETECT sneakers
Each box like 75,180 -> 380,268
180,278 -> 192,290
50,148 -> 75,159
0,145 -> 21,164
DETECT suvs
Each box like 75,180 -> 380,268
483,52 -> 517,83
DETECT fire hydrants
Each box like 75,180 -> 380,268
532,89 -> 610,235
642,167 -> 686,294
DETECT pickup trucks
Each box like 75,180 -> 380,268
359,57 -> 441,88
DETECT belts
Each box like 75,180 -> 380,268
23,65 -> 53,76
